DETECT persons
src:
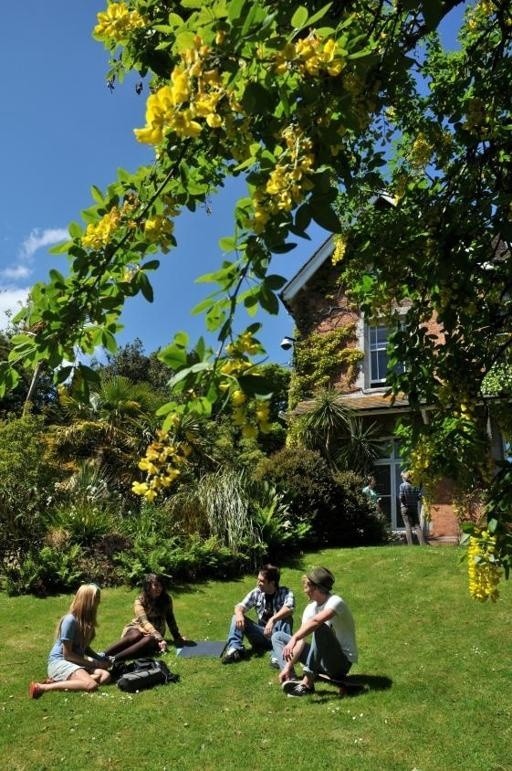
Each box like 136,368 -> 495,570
277,568 -> 358,697
399,471 -> 431,546
362,477 -> 382,513
29,582 -> 112,699
219,566 -> 293,672
87,573 -> 196,666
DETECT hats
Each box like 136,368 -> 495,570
307,566 -> 335,592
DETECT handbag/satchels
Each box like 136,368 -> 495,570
118,666 -> 165,693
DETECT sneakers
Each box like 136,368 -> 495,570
280,681 -> 317,697
29,676 -> 51,699
220,646 -> 244,663
